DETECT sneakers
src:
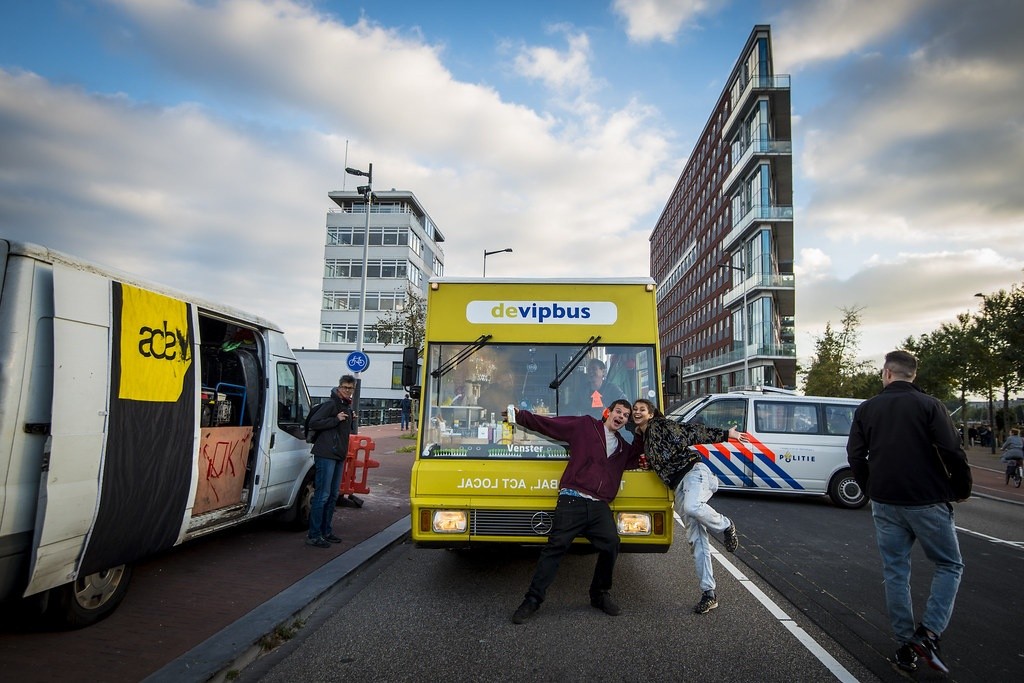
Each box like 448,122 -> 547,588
895,646 -> 918,670
512,596 -> 540,624
724,517 -> 738,552
305,534 -> 332,548
907,626 -> 949,674
323,532 -> 341,543
590,592 -> 622,615
696,593 -> 718,613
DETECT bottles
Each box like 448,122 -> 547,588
490,412 -> 496,428
640,453 -> 650,469
507,404 -> 516,425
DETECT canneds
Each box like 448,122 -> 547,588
491,412 -> 495,424
640,453 -> 648,469
507,404 -> 516,426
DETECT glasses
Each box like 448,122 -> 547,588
340,386 -> 354,392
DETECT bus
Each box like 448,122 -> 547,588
400,276 -> 685,554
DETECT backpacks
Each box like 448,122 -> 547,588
304,400 -> 340,443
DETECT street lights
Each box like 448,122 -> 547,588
716,263 -> 751,385
483,248 -> 513,277
974,293 -> 998,454
344,161 -> 383,433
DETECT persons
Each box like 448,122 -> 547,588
450,385 -> 466,406
304,375 -> 359,549
999,427 -> 1024,480
806,407 -> 832,434
501,398 -> 644,625
400,394 -> 410,432
561,358 -> 631,421
477,368 -> 518,421
847,351 -> 973,674
631,398 -> 750,614
955,421 -> 998,447
757,406 -> 764,431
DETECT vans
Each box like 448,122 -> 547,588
665,382 -> 874,510
0,237 -> 321,631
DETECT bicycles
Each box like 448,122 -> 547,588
1000,449 -> 1023,488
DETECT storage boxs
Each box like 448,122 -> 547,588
477,420 -> 513,445
